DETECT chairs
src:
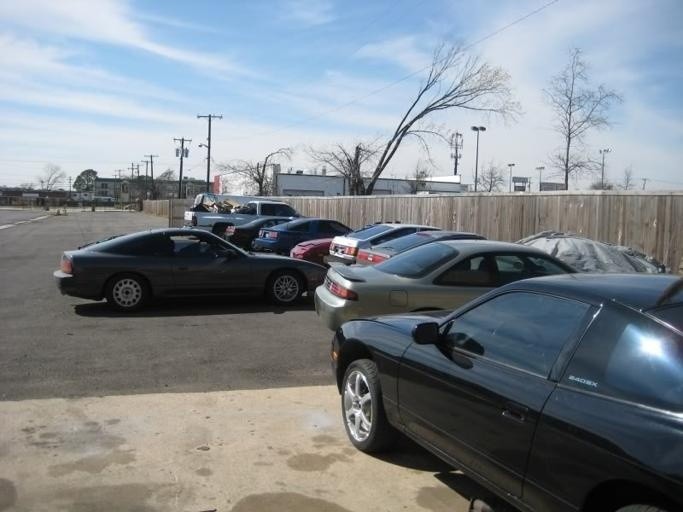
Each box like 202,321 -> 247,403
479,258 -> 499,286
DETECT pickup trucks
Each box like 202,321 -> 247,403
184,200 -> 303,235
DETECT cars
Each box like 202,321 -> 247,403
54,228 -> 327,306
314,239 -> 580,331
331,272 -> 683,512
224,216 -> 486,263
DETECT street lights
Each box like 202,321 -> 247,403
508,164 -> 515,193
199,143 -> 210,192
472,127 -> 485,192
536,167 -> 545,191
600,149 -> 611,190
